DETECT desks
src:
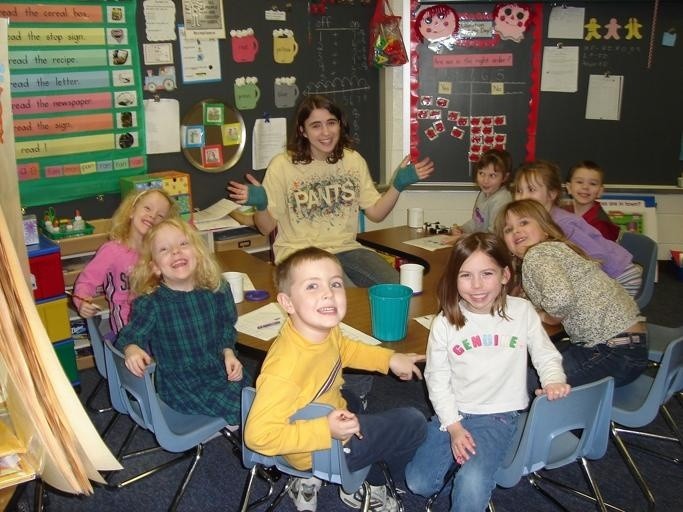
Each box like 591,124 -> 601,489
195,224 -> 586,390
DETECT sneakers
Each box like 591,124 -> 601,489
285,477 -> 322,512
339,480 -> 400,512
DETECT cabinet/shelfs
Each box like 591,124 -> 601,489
119,169 -> 193,229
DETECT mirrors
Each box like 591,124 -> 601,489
179,94 -> 245,174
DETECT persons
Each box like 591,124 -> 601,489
65,189 -> 193,341
493,198 -> 650,412
559,160 -> 622,243
223,96 -> 440,289
241,245 -> 430,511
445,147 -> 513,236
507,155 -> 647,300
110,216 -> 281,482
402,222 -> 571,511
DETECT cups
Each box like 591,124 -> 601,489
399,263 -> 425,295
220,271 -> 243,304
368,283 -> 413,341
406,207 -> 424,228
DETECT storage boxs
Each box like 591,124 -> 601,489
27,233 -> 83,393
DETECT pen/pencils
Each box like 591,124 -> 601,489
257,321 -> 280,329
453,224 -> 459,230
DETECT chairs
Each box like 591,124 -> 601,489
597,337 -> 682,510
420,374 -> 617,511
97,334 -> 275,511
639,321 -> 682,404
79,307 -> 164,460
616,232 -> 658,309
236,384 -> 406,512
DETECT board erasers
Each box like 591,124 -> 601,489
677,177 -> 683,188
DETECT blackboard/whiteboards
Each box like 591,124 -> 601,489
21,0 -> 387,231
401,0 -> 683,195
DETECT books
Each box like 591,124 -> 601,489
190,199 -> 241,231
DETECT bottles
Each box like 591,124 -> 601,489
44,209 -> 84,233
